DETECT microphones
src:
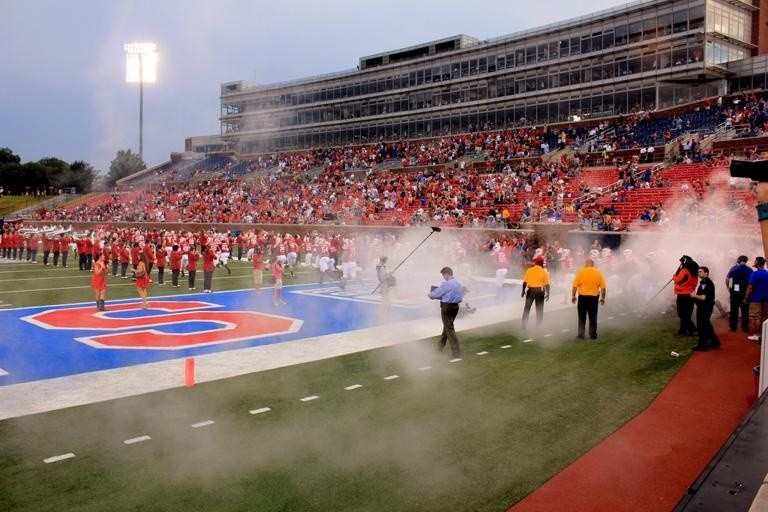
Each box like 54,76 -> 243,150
431,227 -> 441,233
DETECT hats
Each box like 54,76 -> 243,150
533,256 -> 543,263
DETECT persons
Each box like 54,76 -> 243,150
0,92 -> 768,359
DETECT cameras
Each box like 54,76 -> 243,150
729,159 -> 768,183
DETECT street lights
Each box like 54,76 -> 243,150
124,40 -> 160,161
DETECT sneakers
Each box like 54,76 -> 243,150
747,336 -> 758,341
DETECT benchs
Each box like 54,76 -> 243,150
56,100 -> 768,250
